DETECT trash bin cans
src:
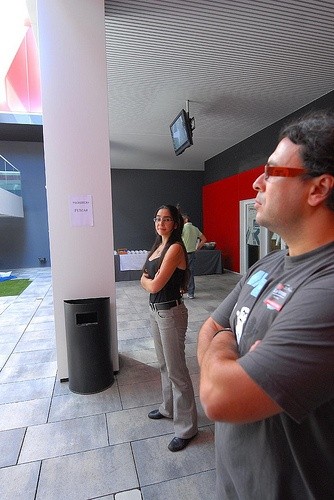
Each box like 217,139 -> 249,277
63,296 -> 114,395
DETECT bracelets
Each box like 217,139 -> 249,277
213,328 -> 232,338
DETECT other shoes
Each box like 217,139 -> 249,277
187,293 -> 194,299
148,409 -> 173,419
168,432 -> 199,452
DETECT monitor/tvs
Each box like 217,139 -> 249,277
170,109 -> 194,156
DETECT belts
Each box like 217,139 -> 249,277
150,300 -> 181,311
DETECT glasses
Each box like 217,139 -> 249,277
153,217 -> 175,223
263,164 -> 313,181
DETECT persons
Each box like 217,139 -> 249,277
179,213 -> 206,300
141,205 -> 199,452
195,112 -> 334,500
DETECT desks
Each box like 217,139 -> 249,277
115,248 -> 224,282
